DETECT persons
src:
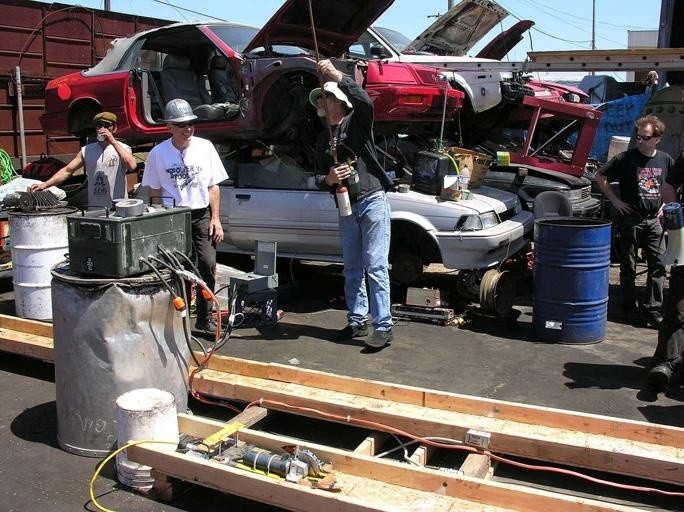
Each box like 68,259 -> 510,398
26,111 -> 138,212
140,98 -> 231,335
649,149 -> 684,390
594,114 -> 677,330
644,67 -> 660,95
309,58 -> 395,350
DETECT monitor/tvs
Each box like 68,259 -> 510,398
410,150 -> 460,195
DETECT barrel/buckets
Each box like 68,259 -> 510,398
114,388 -> 180,489
530,217 -> 614,346
8,206 -> 79,320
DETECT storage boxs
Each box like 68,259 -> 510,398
443,146 -> 495,190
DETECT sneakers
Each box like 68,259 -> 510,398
333,323 -> 369,341
619,307 -> 647,328
639,310 -> 664,330
646,362 -> 675,387
193,317 -> 225,336
364,331 -> 394,348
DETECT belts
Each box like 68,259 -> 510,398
631,212 -> 658,219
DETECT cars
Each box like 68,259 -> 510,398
374,122 -> 604,219
133,139 -> 535,270
39,1 -> 465,144
474,19 -> 591,122
349,0 -> 509,113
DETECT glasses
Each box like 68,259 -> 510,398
170,121 -> 196,129
316,93 -> 333,99
97,123 -> 116,128
635,134 -> 657,142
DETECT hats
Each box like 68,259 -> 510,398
156,98 -> 199,122
309,79 -> 352,108
92,112 -> 118,125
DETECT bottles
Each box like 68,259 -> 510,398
460,163 -> 470,191
